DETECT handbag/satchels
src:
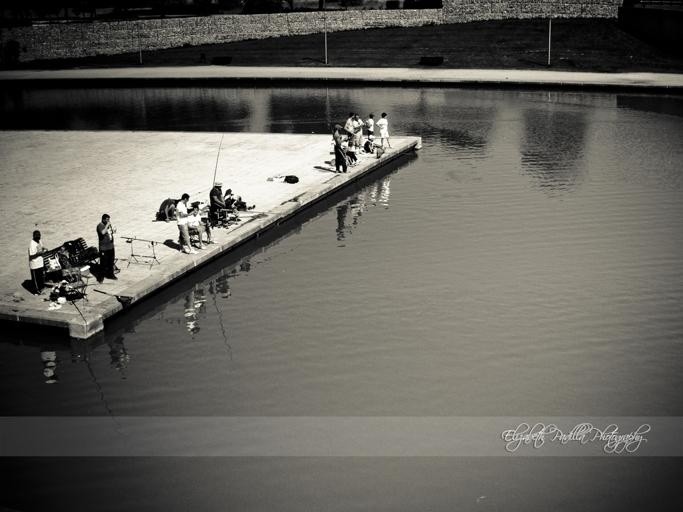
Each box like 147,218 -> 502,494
48,256 -> 62,270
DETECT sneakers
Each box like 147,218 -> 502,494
180,238 -> 217,254
107,274 -> 117,279
98,274 -> 104,283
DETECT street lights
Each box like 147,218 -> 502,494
548,15 -> 553,65
133,22 -> 143,65
318,17 -> 327,64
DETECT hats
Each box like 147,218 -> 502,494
214,182 -> 222,187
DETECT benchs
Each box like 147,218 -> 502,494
40,237 -> 90,284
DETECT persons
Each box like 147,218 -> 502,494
159,181 -> 240,254
334,112 -> 392,172
28,231 -> 47,296
41,346 -> 58,386
183,275 -> 232,335
109,337 -> 129,380
336,177 -> 391,241
97,214 -> 118,283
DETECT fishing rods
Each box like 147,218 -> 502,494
213,123 -> 227,187
73,282 -> 114,289
219,209 -> 287,214
372,152 -> 413,156
120,237 -> 163,246
